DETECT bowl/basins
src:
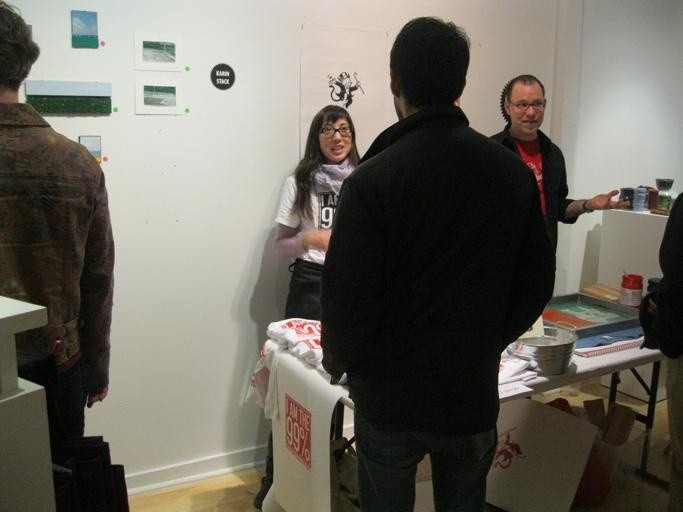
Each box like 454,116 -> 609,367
507,322 -> 578,377
656,179 -> 674,191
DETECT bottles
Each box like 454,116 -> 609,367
647,276 -> 660,293
619,273 -> 645,307
658,191 -> 671,211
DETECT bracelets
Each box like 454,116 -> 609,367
583,199 -> 591,213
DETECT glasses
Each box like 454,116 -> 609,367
511,101 -> 546,110
320,127 -> 352,137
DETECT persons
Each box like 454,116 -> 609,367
0,0 -> 116,440
646,189 -> 682,511
319,14 -> 554,511
489,73 -> 632,245
250,104 -> 361,512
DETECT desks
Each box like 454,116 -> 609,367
251,294 -> 669,511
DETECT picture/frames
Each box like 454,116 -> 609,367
134,79 -> 183,117
131,32 -> 183,74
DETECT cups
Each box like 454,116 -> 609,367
633,187 -> 649,211
647,188 -> 659,210
